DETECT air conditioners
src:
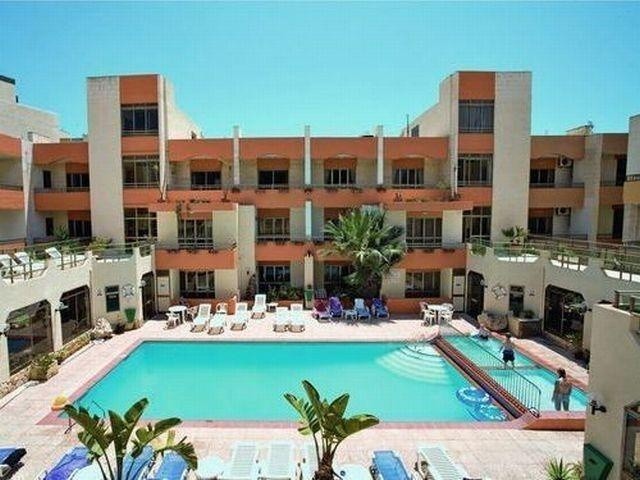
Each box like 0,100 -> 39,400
558,157 -> 572,168
555,207 -> 570,216
496,224 -> 533,256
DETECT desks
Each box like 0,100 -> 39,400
168,306 -> 193,325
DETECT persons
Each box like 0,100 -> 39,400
479,324 -> 491,340
551,368 -> 572,411
498,333 -> 517,369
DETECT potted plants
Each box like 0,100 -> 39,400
124,308 -> 136,330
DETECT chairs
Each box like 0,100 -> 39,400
368,451 -> 417,480
419,301 -> 454,327
33,443 -> 95,480
191,294 -> 307,334
217,440 -> 337,480
188,303 -> 198,321
311,296 -> 390,322
153,446 -> 191,480
418,448 -> 469,479
109,443 -> 153,480
0,449 -> 28,480
163,313 -> 179,328
0,247 -> 87,282
